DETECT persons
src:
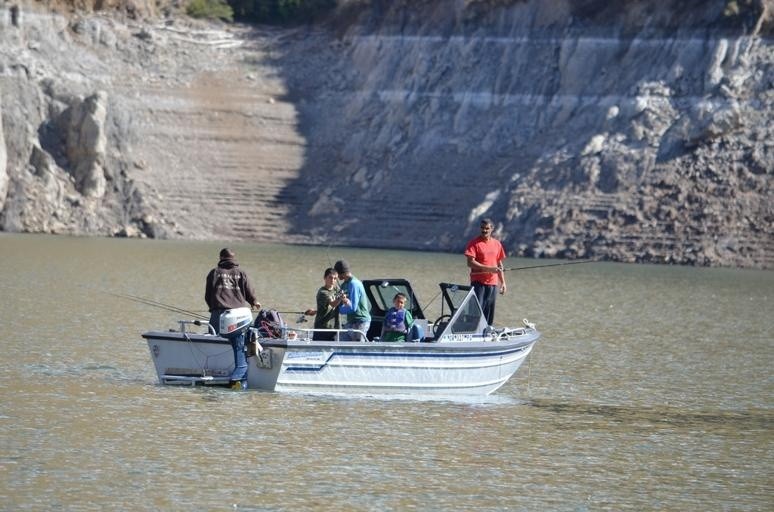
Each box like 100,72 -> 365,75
377,293 -> 414,343
312,268 -> 342,341
204,247 -> 261,335
332,260 -> 372,341
464,218 -> 508,327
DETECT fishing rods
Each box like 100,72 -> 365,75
326,251 -> 344,299
99,290 -> 210,321
470,254 -> 668,276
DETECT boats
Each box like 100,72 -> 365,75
141,279 -> 538,397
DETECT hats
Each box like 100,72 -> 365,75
334,261 -> 349,274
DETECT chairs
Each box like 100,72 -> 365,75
412,323 -> 425,342
